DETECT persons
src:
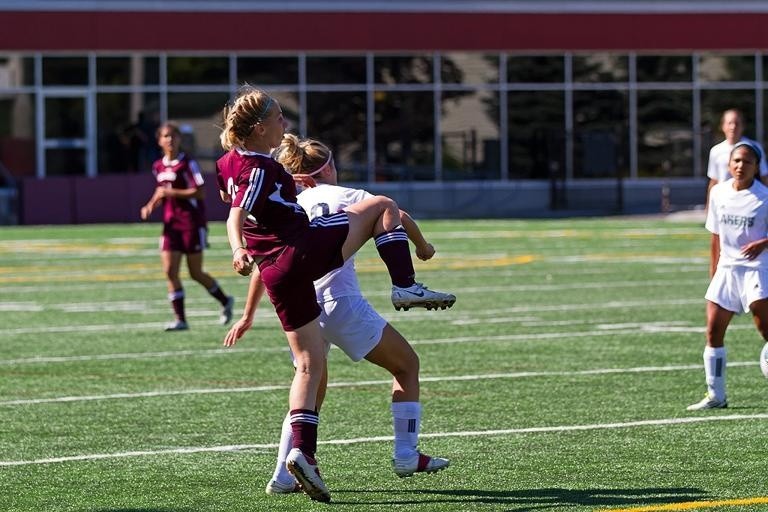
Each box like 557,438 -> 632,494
708,107 -> 768,281
216,81 -> 457,503
683,144 -> 768,413
224,134 -> 452,495
140,122 -> 235,332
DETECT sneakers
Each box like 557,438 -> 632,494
393,451 -> 449,477
391,283 -> 456,310
686,396 -> 727,411
286,448 -> 330,502
222,296 -> 233,324
166,321 -> 187,331
265,479 -> 299,493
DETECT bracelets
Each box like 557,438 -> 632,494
231,246 -> 245,255
